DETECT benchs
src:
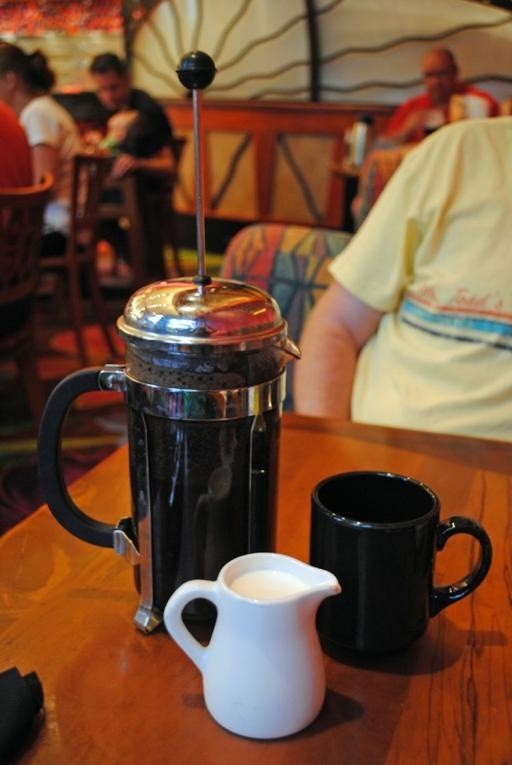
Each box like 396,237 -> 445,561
219,222 -> 353,413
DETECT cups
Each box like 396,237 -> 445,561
307,468 -> 495,657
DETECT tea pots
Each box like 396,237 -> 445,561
33,273 -> 303,636
161,549 -> 345,743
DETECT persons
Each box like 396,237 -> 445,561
0,41 -> 88,260
292,115 -> 512,442
0,100 -> 33,339
83,54 -> 178,280
383,48 -> 501,146
